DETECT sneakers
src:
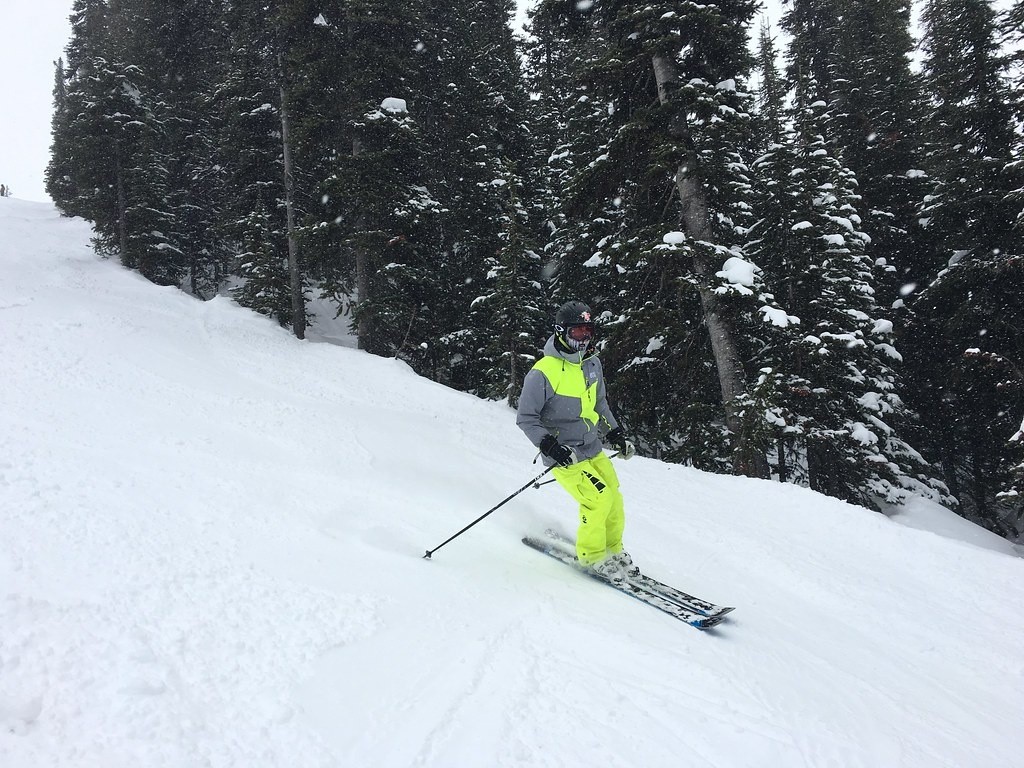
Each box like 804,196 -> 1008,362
611,550 -> 641,580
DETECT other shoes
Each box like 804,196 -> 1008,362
587,556 -> 623,582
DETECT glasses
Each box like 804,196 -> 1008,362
555,323 -> 594,342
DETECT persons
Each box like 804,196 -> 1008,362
516,301 -> 639,583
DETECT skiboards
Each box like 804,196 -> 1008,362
520,527 -> 739,631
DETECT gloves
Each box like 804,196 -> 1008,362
606,427 -> 636,460
540,434 -> 577,468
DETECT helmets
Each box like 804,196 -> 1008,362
554,301 -> 596,364
